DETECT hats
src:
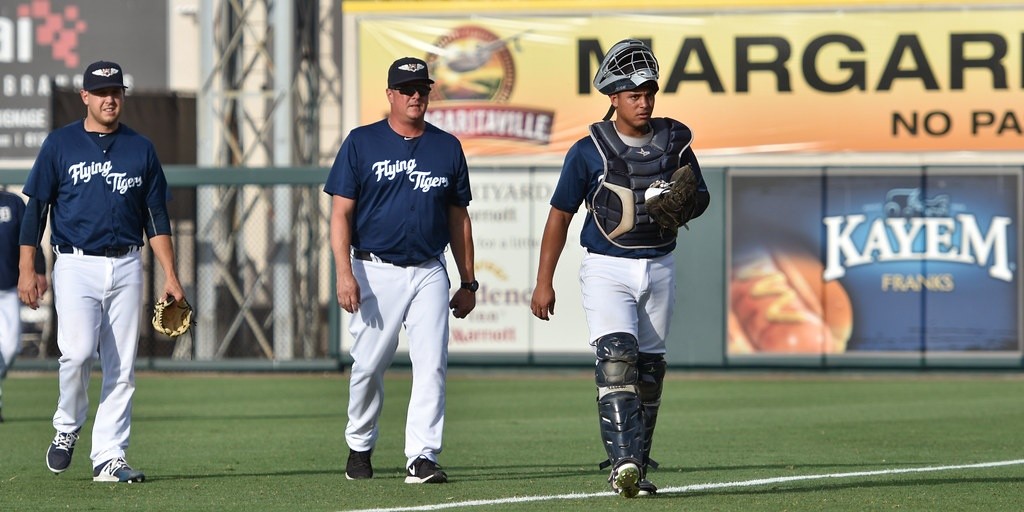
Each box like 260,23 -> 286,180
388,57 -> 435,88
83,60 -> 128,91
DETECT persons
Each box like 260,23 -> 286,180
0,191 -> 51,422
14,63 -> 189,482
329,56 -> 480,484
531,37 -> 710,498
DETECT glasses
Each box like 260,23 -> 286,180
389,85 -> 431,97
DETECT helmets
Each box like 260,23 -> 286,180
593,39 -> 660,95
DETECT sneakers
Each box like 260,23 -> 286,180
612,462 -> 650,498
405,455 -> 448,483
46,427 -> 81,473
345,448 -> 373,480
93,458 -> 145,483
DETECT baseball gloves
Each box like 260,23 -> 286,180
644,164 -> 698,231
152,296 -> 193,337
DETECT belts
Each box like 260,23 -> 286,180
54,245 -> 139,258
354,250 -> 426,267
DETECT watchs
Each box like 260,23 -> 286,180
461,280 -> 478,292
